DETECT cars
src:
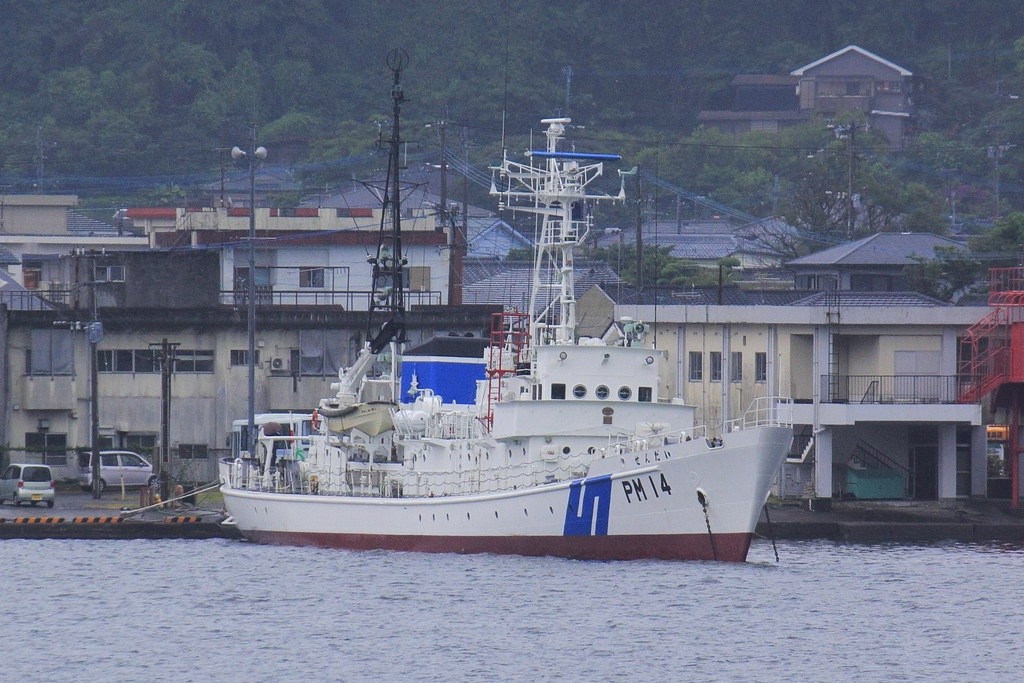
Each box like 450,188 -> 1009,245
0,463 -> 55,508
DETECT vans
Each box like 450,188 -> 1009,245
76,451 -> 159,492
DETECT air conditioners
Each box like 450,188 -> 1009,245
271,356 -> 287,371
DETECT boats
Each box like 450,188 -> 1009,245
219,118 -> 796,563
